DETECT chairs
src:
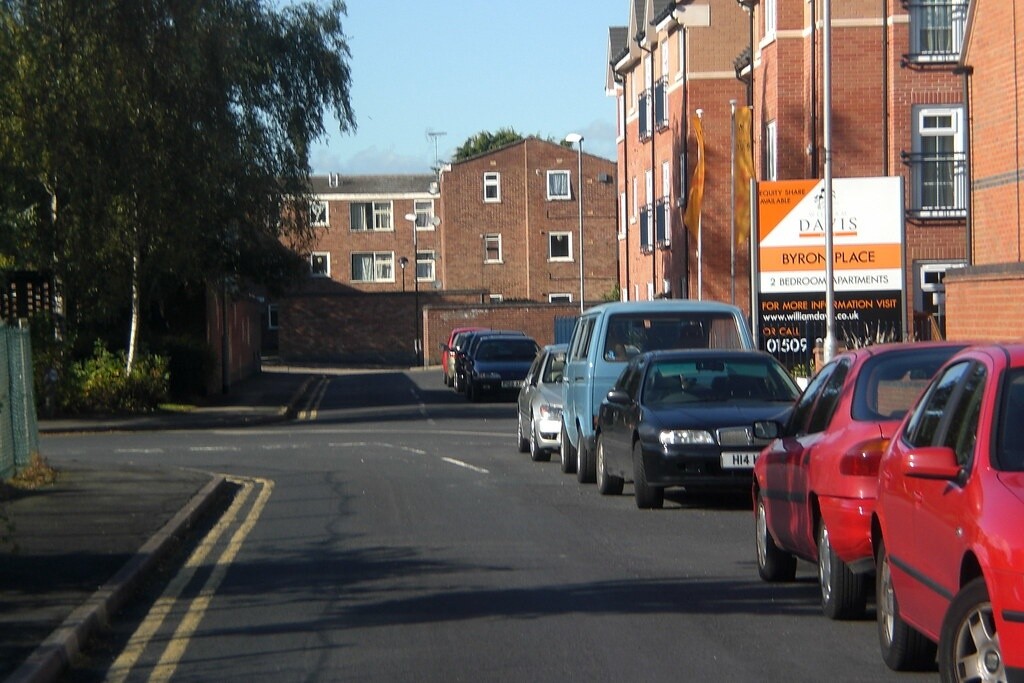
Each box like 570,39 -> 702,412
660,375 -> 772,403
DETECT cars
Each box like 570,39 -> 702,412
514,343 -> 571,462
871,340 -> 1024,682
437,327 -> 528,387
596,347 -> 808,510
460,334 -> 544,402
751,340 -> 1024,621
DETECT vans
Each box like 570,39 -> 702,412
559,298 -> 756,484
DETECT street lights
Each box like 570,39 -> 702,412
404,214 -> 418,367
397,257 -> 408,294
564,133 -> 586,311
